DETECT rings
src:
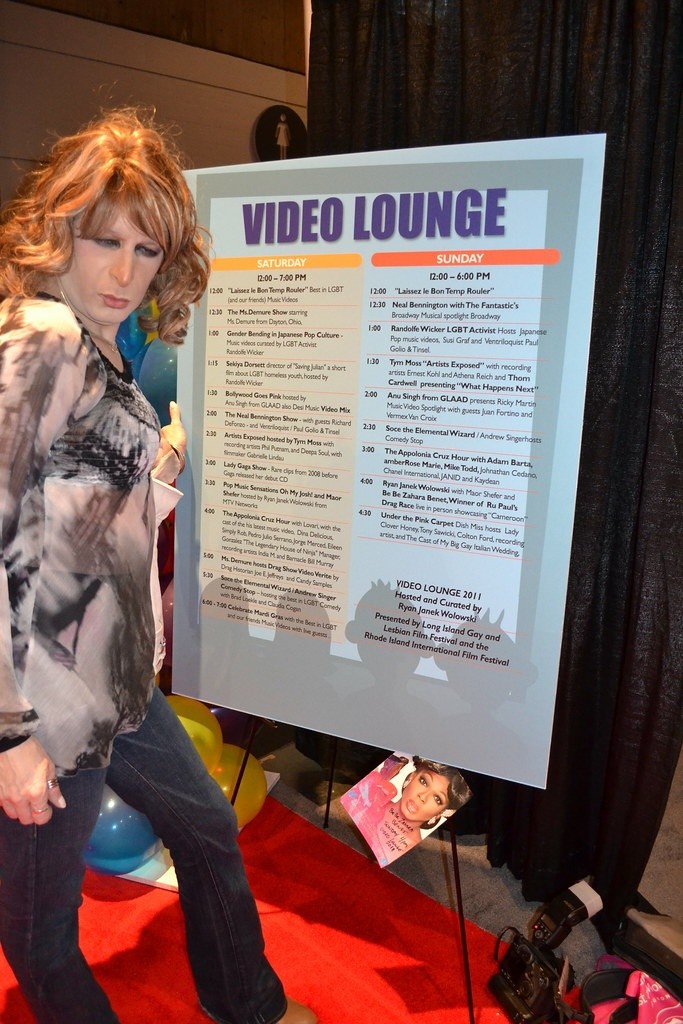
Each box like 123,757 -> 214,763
30,806 -> 48,814
46,777 -> 59,790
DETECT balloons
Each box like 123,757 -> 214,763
155,673 -> 267,827
83,783 -> 163,876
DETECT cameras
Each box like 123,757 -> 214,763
486,880 -> 603,1024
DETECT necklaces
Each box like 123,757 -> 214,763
59,286 -> 119,354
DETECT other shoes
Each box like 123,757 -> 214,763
275,998 -> 317,1024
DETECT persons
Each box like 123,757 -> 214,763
340,756 -> 473,868
0,109 -> 319,1024
